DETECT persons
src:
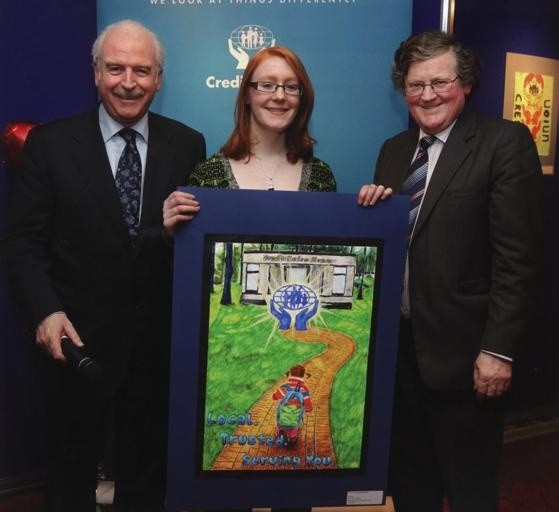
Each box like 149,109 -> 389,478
160,42 -> 395,511
0,17 -> 207,511
372,30 -> 542,510
270,364 -> 314,446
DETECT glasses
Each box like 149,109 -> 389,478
248,81 -> 303,96
404,75 -> 458,96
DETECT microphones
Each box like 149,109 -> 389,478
61,340 -> 104,380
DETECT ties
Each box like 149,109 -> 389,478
115,129 -> 142,248
400,135 -> 437,241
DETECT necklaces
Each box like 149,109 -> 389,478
255,151 -> 286,190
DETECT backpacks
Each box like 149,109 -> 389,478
277,384 -> 310,429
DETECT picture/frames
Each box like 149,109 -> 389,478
165,188 -> 410,509
